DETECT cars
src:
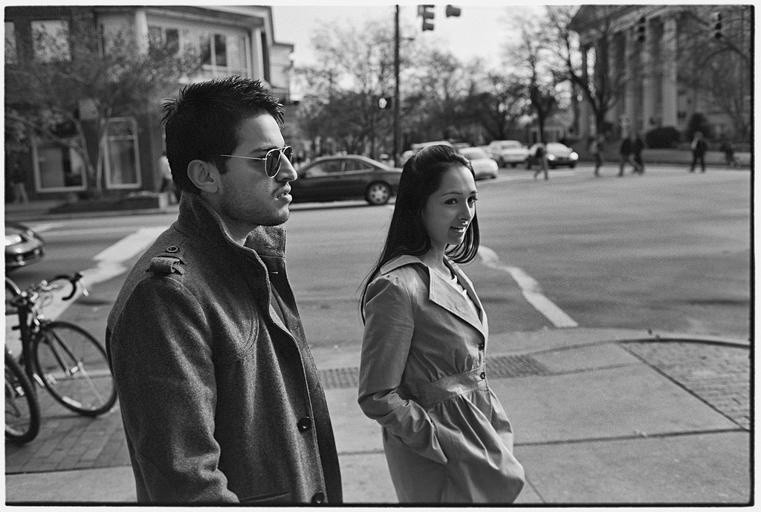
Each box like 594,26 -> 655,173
287,155 -> 404,206
400,139 -> 529,180
525,142 -> 579,169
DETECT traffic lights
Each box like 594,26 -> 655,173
708,12 -> 723,43
379,97 -> 392,110
423,5 -> 434,32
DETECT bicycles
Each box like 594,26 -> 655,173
5,272 -> 117,442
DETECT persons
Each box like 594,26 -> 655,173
630,132 -> 645,175
357,143 -> 527,504
156,152 -> 177,193
718,133 -> 740,167
690,131 -> 707,173
103,75 -> 345,506
617,131 -> 644,176
589,134 -> 607,179
533,146 -> 550,180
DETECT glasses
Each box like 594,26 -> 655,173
220,146 -> 292,178
